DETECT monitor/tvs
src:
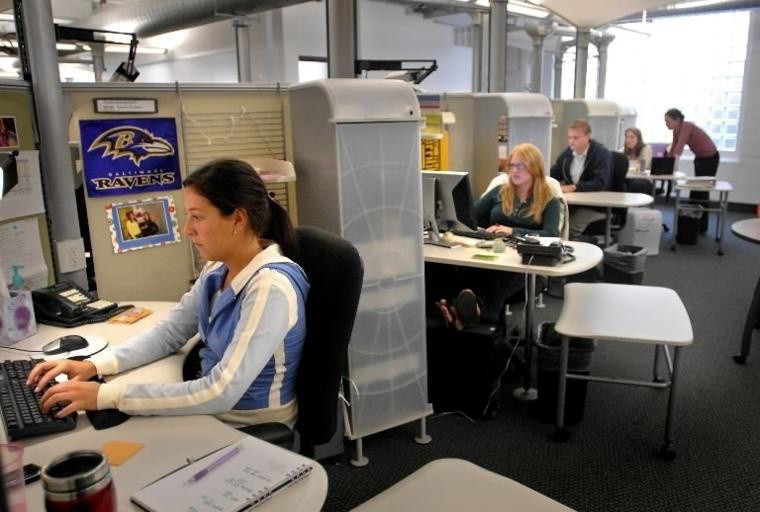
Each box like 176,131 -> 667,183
421,169 -> 477,250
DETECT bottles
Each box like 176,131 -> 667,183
493,238 -> 506,253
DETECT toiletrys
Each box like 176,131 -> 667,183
7,265 -> 30,296
0,270 -> 38,345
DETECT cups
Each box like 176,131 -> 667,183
0,443 -> 118,512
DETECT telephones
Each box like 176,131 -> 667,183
517,234 -> 563,267
31,280 -> 118,325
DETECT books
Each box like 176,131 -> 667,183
129,437 -> 313,512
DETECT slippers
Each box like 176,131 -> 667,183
456,290 -> 474,320
435,299 -> 457,327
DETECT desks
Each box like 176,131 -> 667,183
424,227 -> 603,402
562,192 -> 657,249
555,281 -> 696,463
342,456 -> 581,511
670,177 -> 733,257
0,300 -> 328,512
732,217 -> 760,366
621,171 -> 679,208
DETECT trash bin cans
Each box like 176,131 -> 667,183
536,321 -> 596,416
677,204 -> 703,245
603,243 -> 648,285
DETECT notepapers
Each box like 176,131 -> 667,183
98,440 -> 144,466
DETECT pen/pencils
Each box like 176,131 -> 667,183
186,444 -> 243,482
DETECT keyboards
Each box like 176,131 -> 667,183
440,225 -> 509,242
0,357 -> 78,445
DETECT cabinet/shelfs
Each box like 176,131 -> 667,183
564,98 -> 620,153
291,77 -> 433,468
423,92 -> 556,199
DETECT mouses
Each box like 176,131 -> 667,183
42,334 -> 88,354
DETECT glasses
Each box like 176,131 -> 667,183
509,163 -> 525,170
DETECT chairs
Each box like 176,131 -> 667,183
181,224 -> 364,456
479,174 -> 570,337
649,144 -> 666,207
582,152 -> 630,248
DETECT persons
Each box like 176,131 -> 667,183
549,119 -> 617,238
125,210 -> 143,239
664,108 -> 720,233
143,211 -> 159,235
25,156 -> 313,455
615,127 -> 654,194
434,142 -> 568,332
